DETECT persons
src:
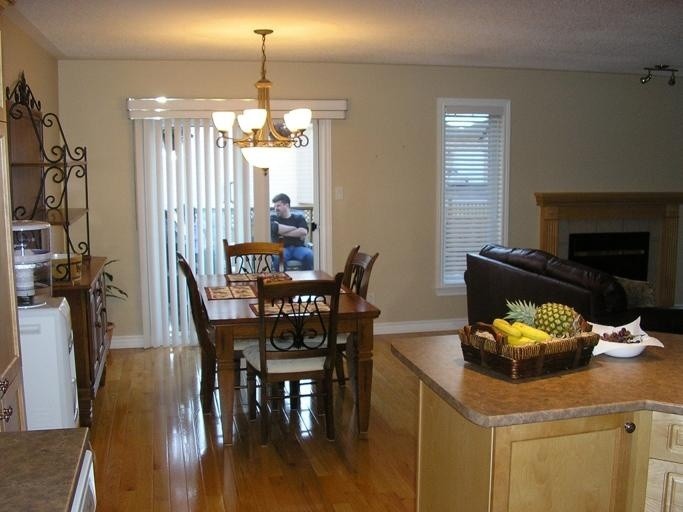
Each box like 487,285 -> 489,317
270,194 -> 314,273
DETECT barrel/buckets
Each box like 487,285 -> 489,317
11,220 -> 53,307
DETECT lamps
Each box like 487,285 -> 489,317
213,29 -> 313,170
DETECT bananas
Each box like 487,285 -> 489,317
513,322 -> 551,341
508,336 -> 529,347
494,318 -> 522,338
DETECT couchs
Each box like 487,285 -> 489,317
463,239 -> 683,340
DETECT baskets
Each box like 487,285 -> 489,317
457,321 -> 599,381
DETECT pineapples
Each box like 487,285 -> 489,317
502,298 -> 592,337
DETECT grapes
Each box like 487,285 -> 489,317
598,327 -> 641,344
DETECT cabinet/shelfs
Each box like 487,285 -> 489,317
5,70 -> 107,430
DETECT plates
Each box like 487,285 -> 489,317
603,345 -> 647,358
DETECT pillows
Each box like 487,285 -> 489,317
612,272 -> 656,308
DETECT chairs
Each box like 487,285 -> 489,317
174,251 -> 245,407
343,243 -> 380,299
243,271 -> 346,448
220,237 -> 285,271
